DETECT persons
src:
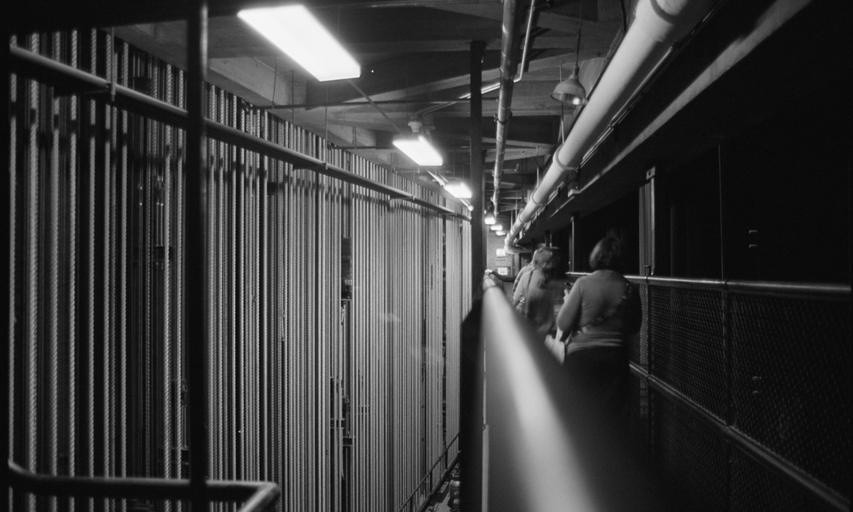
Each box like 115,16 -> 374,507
511,247 -> 544,293
554,233 -> 643,436
509,245 -> 557,342
489,257 -> 531,283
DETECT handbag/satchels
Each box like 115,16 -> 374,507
545,328 -> 572,366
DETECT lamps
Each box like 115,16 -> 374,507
549,0 -> 586,106
